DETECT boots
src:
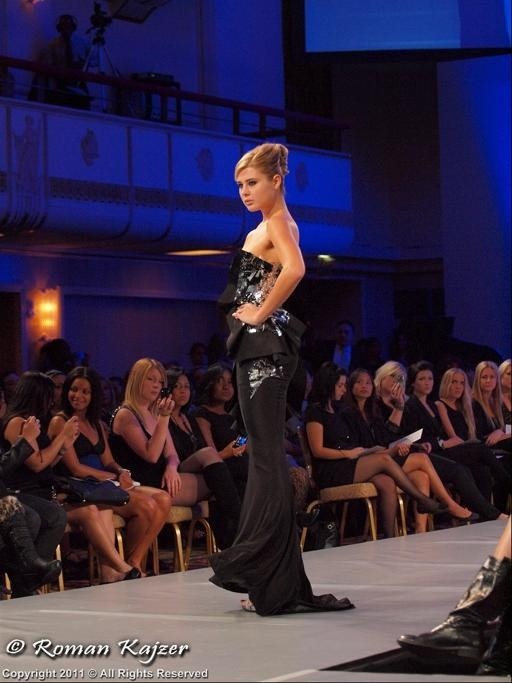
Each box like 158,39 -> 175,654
1,542 -> 42,599
398,553 -> 511,665
477,613 -> 512,675
0,494 -> 61,598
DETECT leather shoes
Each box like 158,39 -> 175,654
295,507 -> 320,533
127,567 -> 141,578
452,510 -> 480,520
417,498 -> 450,514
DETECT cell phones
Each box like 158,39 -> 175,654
161,388 -> 172,398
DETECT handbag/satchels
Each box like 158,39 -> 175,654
306,520 -> 338,549
69,474 -> 130,504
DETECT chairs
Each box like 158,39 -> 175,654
0,421 -> 437,600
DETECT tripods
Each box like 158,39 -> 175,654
74,35 -> 137,118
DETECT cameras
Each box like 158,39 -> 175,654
393,374 -> 404,384
234,434 -> 248,447
17,419 -> 40,437
74,352 -> 86,359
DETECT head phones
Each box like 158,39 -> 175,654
56,14 -> 78,32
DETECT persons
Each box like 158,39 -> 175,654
1,318 -> 511,683
207,142 -> 359,618
35,13 -> 93,108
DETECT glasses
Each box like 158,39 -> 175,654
175,382 -> 191,389
